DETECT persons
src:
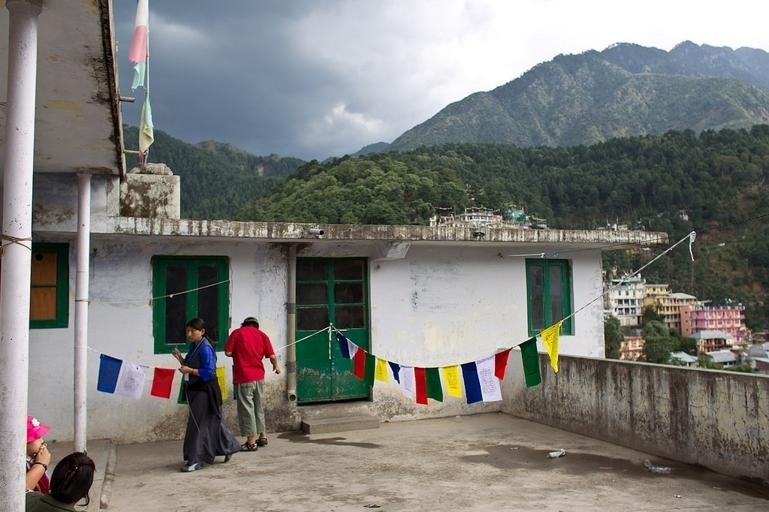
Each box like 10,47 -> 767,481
171,317 -> 245,473
26,414 -> 51,494
224,316 -> 281,453
25,450 -> 96,512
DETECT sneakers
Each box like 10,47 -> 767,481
182,463 -> 200,472
240,438 -> 267,450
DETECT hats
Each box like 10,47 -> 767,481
27,416 -> 48,444
244,317 -> 259,327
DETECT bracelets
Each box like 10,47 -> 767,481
33,461 -> 47,472
190,368 -> 194,374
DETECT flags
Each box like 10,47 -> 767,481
151,367 -> 177,400
96,354 -> 122,393
215,368 -> 230,402
335,318 -> 565,407
126,0 -> 155,159
115,360 -> 149,399
177,372 -> 188,406
